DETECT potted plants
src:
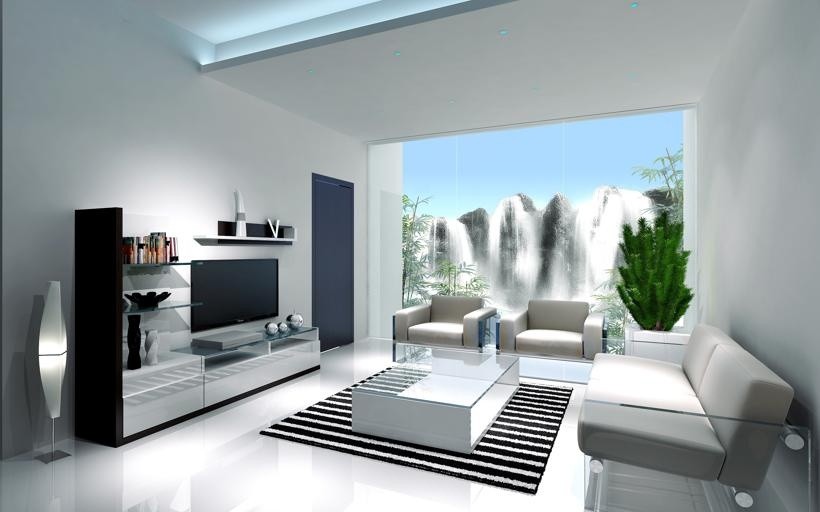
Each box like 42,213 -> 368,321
615,210 -> 695,366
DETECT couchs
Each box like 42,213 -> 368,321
577,322 -> 795,492
393,295 -> 497,350
498,299 -> 609,360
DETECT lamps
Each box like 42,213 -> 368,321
35,280 -> 72,465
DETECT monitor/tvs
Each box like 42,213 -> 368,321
189,258 -> 280,350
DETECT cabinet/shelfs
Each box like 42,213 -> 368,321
75,206 -> 321,448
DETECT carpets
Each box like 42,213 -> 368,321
260,366 -> 573,495
